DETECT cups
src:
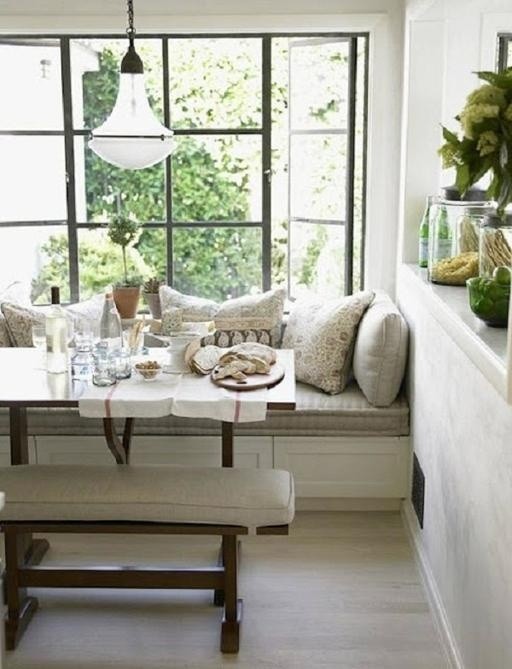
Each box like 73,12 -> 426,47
122,331 -> 147,354
109,349 -> 132,379
133,361 -> 169,381
91,353 -> 118,387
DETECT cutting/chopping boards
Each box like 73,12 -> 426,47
210,360 -> 285,389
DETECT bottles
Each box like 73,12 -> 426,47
435,203 -> 453,262
419,194 -> 430,267
45,285 -> 70,372
100,291 -> 124,347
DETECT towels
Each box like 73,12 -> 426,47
79,369 -> 268,423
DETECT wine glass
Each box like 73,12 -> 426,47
31,324 -> 46,369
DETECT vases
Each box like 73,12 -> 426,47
479,224 -> 512,274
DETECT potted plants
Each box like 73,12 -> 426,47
143,279 -> 166,320
109,214 -> 142,318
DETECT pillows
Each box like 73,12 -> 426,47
353,293 -> 408,409
2,300 -> 35,347
280,291 -> 375,396
159,285 -> 286,349
30,284 -> 123,348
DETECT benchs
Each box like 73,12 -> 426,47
0,463 -> 294,657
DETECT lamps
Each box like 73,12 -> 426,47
86,28 -> 181,171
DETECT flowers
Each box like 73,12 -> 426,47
439,70 -> 511,217
